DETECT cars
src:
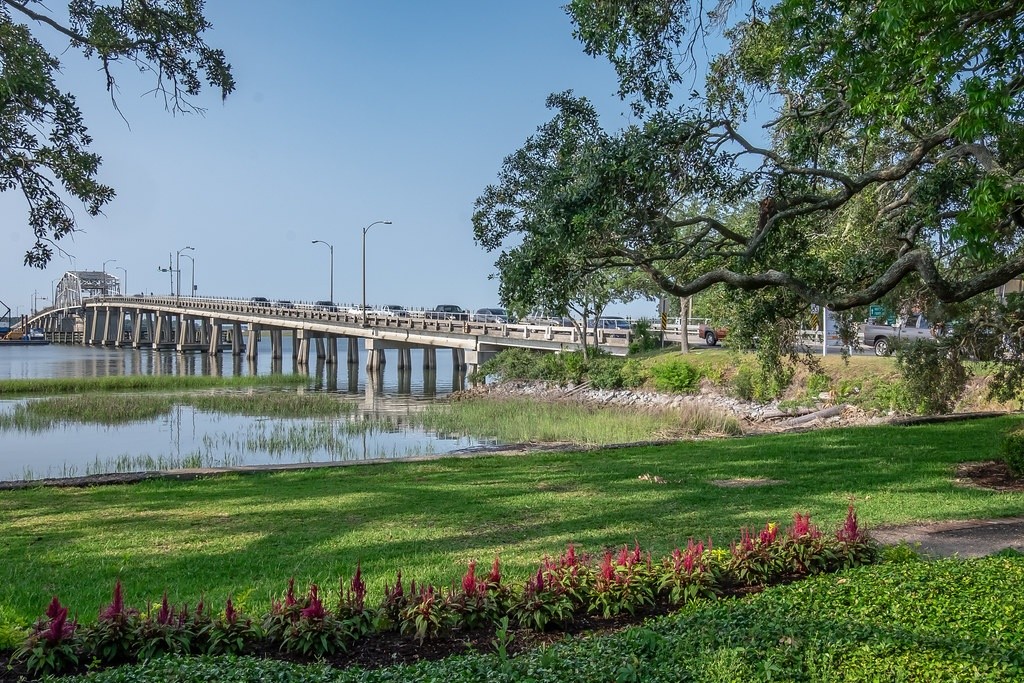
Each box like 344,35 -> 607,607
249,297 -> 270,307
312,301 -> 336,312
528,310 -> 574,332
699,321 -> 729,346
272,301 -> 294,310
579,316 -> 636,337
348,305 -> 377,319
425,305 -> 468,321
473,308 -> 517,324
381,306 -> 409,318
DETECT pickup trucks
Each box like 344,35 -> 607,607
864,313 -> 956,357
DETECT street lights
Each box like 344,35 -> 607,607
362,220 -> 392,329
52,279 -> 57,310
312,240 -> 333,303
102,259 -> 127,303
175,246 -> 195,311
